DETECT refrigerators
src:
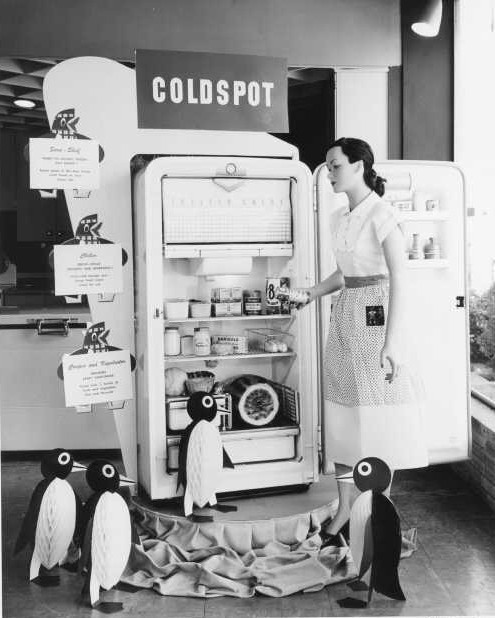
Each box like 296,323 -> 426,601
136,156 -> 474,506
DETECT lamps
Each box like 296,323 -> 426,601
411,1 -> 445,39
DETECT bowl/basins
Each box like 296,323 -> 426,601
211,343 -> 233,356
183,371 -> 215,394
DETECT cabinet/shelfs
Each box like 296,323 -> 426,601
0,131 -> 17,211
2,292 -> 82,307
15,132 -> 74,243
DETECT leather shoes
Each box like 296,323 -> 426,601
320,517 -> 350,548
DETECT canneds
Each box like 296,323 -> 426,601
243,289 -> 262,315
194,327 -> 210,355
165,327 -> 181,355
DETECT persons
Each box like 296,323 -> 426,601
278,136 -> 409,551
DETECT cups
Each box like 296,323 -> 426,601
425,200 -> 439,212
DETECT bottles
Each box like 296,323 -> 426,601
423,236 -> 440,260
164,327 -> 181,355
409,233 -> 426,260
180,335 -> 193,355
192,325 -> 210,356
